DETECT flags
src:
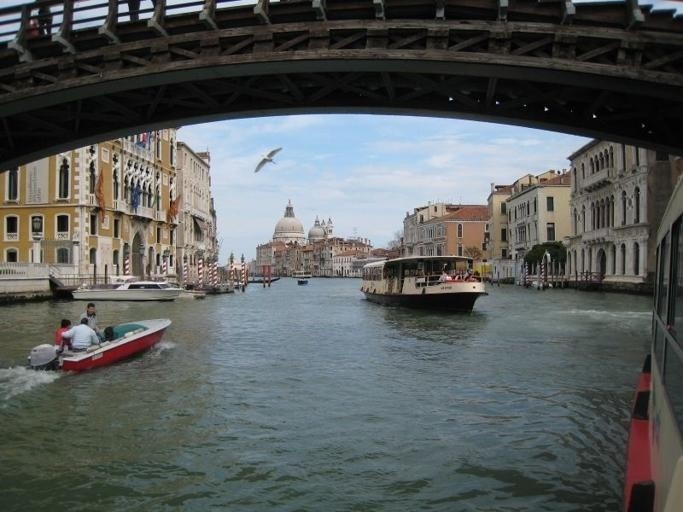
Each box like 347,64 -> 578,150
129,182 -> 141,214
94,168 -> 105,223
168,194 -> 181,222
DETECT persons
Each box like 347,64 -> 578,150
60,317 -> 99,352
439,267 -> 481,283
78,303 -> 101,342
22,7 -> 52,39
53,319 -> 72,351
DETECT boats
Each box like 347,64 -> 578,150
361,255 -> 489,311
248,277 -> 280,283
298,279 -> 308,285
625,174 -> 682,511
71,280 -> 184,300
292,271 -> 312,279
27,318 -> 172,373
192,288 -> 206,299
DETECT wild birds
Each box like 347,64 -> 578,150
255,146 -> 283,172
